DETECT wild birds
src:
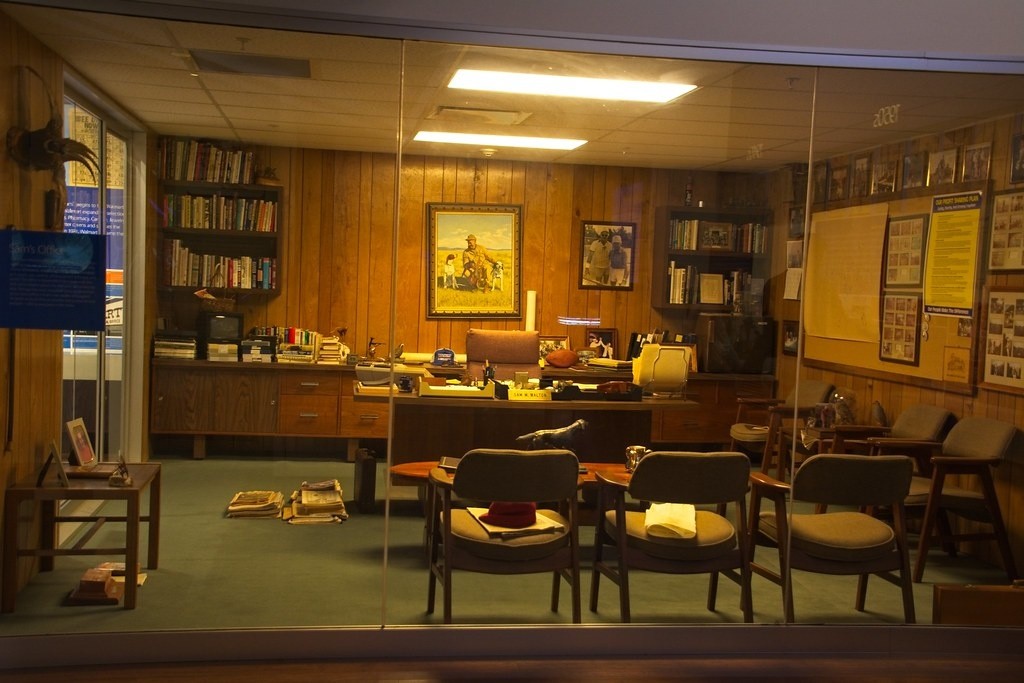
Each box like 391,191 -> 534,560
870,400 -> 887,426
830,397 -> 856,425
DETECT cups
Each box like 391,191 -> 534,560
483,370 -> 496,384
624,446 -> 652,472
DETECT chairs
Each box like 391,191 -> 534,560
813,403 -> 952,514
426,448 -> 582,625
740,454 -> 917,623
730,377 -> 837,475
588,451 -> 753,623
871,416 -> 1020,583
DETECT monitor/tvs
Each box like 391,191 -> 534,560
197,311 -> 243,344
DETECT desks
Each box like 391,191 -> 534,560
391,461 -> 634,569
776,426 -> 873,482
390,385 -> 699,515
4,460 -> 162,610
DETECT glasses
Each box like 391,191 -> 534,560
601,234 -> 609,237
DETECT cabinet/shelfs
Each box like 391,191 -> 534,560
650,206 -> 774,310
158,178 -> 282,294
149,353 -> 775,460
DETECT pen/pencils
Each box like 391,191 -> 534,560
482,359 -> 497,375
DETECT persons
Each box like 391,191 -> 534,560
464,235 -> 498,293
586,231 -> 630,285
77,433 -> 91,460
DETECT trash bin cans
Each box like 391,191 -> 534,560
354,450 -> 377,505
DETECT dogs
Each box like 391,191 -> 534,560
515,418 -> 589,453
443,254 -> 462,290
487,261 -> 504,292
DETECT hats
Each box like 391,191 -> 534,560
611,236 -> 622,245
465,235 -> 476,241
479,500 -> 536,529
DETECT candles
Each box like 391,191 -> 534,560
526,291 -> 537,331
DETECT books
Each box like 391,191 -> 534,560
205,325 -> 341,364
59,466 -> 118,478
71,563 -> 146,606
163,193 -> 277,232
669,219 -> 766,305
675,333 -> 697,342
627,329 -> 669,361
153,335 -> 198,359
438,456 -> 459,473
588,358 -> 632,369
161,137 -> 257,184
227,479 -> 349,524
164,238 -> 277,289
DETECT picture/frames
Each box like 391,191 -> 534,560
813,161 -> 828,204
926,146 -> 959,186
586,327 -> 617,360
788,207 -> 804,237
539,335 -> 571,366
425,202 -> 522,319
851,152 -> 872,198
1009,132 -> 1024,184
828,164 -> 852,201
781,319 -> 799,356
65,418 -> 99,468
578,220 -> 637,291
961,141 -> 993,182
870,160 -> 898,194
902,149 -> 928,190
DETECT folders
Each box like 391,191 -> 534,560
627,332 -> 647,361
362,378 -> 389,386
438,456 -> 461,473
